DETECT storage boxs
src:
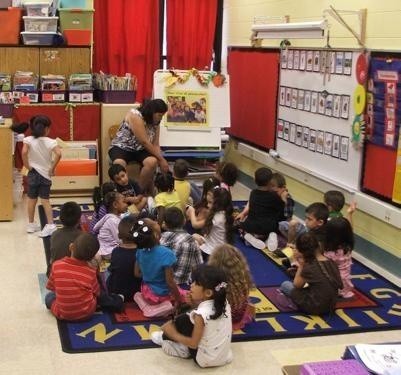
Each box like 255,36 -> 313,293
58,8 -> 95,33
59,0 -> 93,8
22,16 -> 59,32
0,6 -> 25,47
24,2 -> 50,17
19,31 -> 57,47
65,29 -> 91,47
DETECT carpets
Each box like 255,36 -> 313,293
37,203 -> 401,353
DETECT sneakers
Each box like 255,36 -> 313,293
151,331 -> 163,346
267,232 -> 277,251
133,292 -> 148,311
161,340 -> 191,359
143,301 -> 172,318
243,233 -> 265,250
37,223 -> 57,237
25,223 -> 39,233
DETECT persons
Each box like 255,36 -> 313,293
167,96 -> 207,123
107,97 -> 170,193
43,159 -> 254,369
274,190 -> 357,315
235,168 -> 295,252
20,113 -> 63,240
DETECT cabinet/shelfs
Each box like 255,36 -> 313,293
99,102 -> 141,190
13,102 -> 101,193
0,118 -> 14,222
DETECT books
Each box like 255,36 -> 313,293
41,72 -> 66,91
67,73 -> 93,91
94,71 -> 137,91
12,70 -> 40,91
353,342 -> 401,375
0,72 -> 11,91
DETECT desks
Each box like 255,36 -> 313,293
281,365 -> 302,375
